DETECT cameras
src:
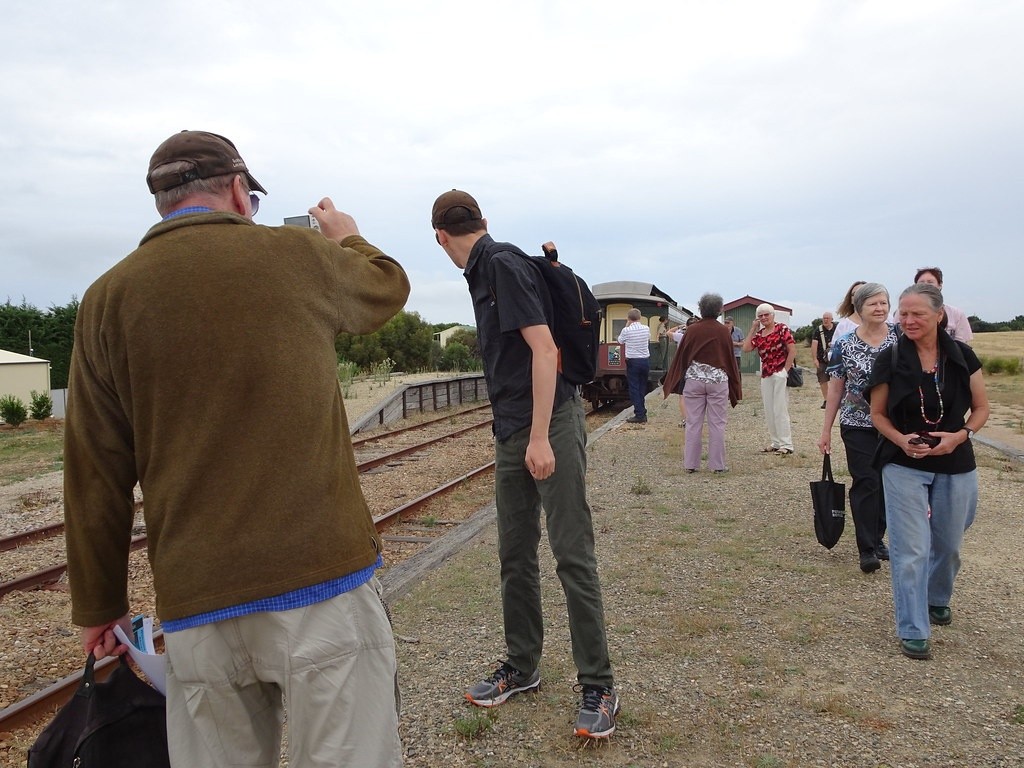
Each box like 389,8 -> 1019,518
284,214 -> 322,235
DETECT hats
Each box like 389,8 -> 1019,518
146,129 -> 268,198
431,188 -> 482,245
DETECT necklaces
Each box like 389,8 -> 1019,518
918,357 -> 944,424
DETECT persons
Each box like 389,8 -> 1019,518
62,130 -> 410,768
662,294 -> 742,473
724,316 -> 744,382
743,303 -> 796,456
812,312 -> 840,409
617,309 -> 650,425
431,188 -> 621,739
819,282 -> 905,574
666,317 -> 699,428
893,267 -> 973,346
828,281 -> 871,362
657,315 -> 668,371
862,284 -> 990,660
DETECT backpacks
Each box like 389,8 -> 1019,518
484,240 -> 601,383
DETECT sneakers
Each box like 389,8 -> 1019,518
571,682 -> 620,737
465,659 -> 539,706
875,541 -> 890,561
860,548 -> 881,574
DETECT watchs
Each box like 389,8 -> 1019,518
961,426 -> 973,439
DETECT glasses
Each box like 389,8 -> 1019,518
758,313 -> 773,318
226,174 -> 260,218
724,321 -> 732,324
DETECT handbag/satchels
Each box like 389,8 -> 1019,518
809,449 -> 845,550
26,639 -> 170,768
785,366 -> 803,387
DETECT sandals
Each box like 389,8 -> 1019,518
774,448 -> 793,455
762,446 -> 778,451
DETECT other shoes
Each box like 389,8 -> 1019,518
687,467 -> 696,473
625,415 -> 647,423
678,420 -> 686,427
715,466 -> 729,473
821,400 -> 826,409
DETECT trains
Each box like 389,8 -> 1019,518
578,280 -> 702,411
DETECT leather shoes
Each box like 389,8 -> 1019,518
903,638 -> 929,660
928,605 -> 952,626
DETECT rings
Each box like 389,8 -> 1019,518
913,453 -> 916,458
